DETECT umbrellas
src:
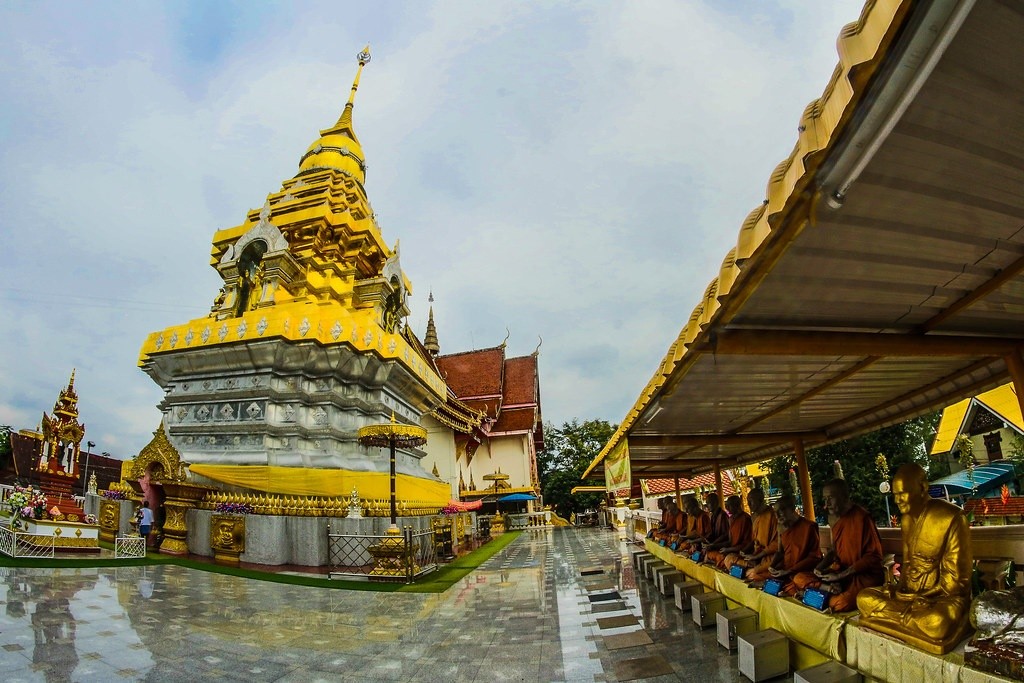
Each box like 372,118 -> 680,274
500,494 -> 539,529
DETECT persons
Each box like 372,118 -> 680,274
384,299 -> 397,333
647,488 -> 822,596
156,500 -> 166,553
794,479 -> 885,612
140,501 -> 155,552
857,463 -> 971,639
246,261 -> 265,311
